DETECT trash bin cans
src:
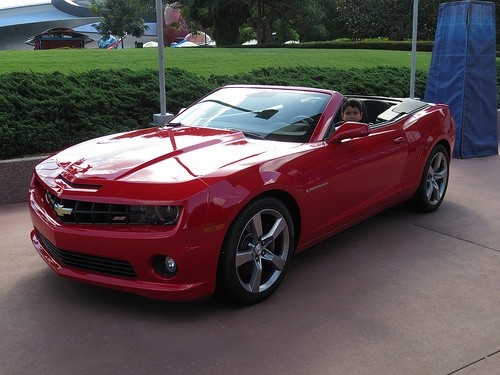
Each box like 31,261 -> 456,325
34,28 -> 85,50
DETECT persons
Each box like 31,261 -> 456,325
341,100 -> 363,124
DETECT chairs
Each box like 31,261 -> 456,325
282,96 -> 390,124
